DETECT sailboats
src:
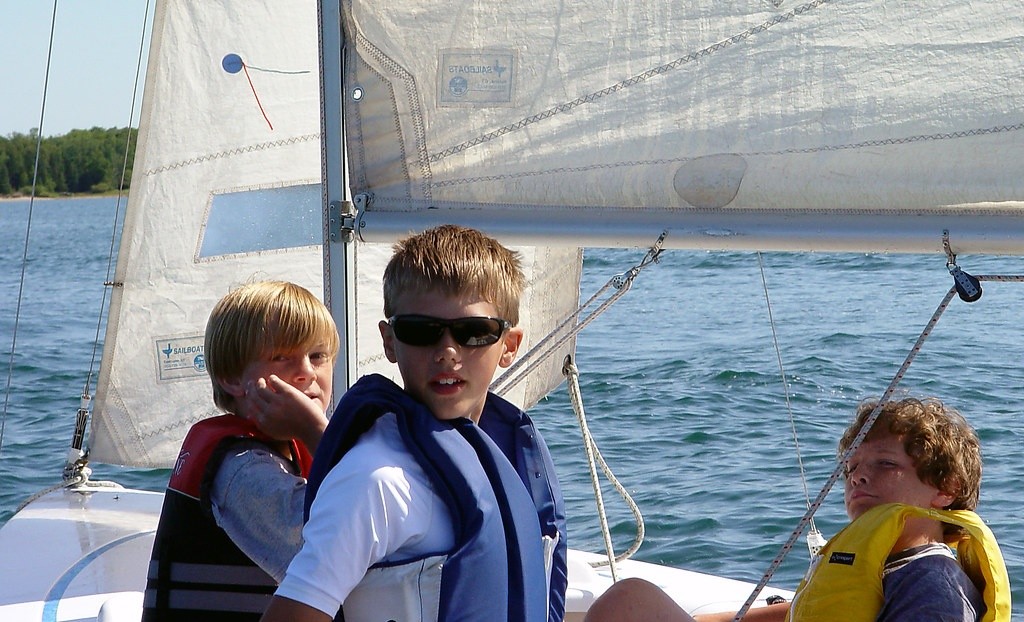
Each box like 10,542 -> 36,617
2,0 -> 1023,622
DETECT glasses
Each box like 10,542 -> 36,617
387,313 -> 509,348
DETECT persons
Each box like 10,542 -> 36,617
141,277 -> 340,622
582,394 -> 1013,622
259,227 -> 567,622
